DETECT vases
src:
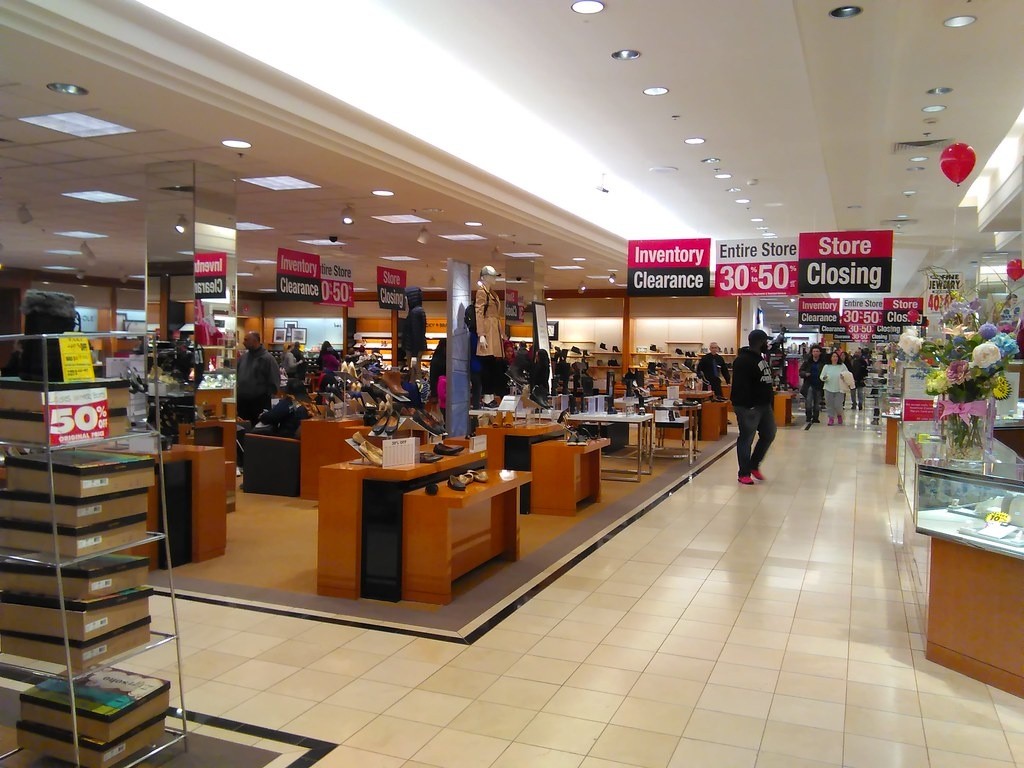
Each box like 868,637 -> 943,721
940,398 -> 995,463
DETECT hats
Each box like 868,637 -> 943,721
748,330 -> 773,340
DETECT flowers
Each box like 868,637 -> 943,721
902,298 -> 1020,402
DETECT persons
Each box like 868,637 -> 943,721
504,342 -> 550,394
402,286 -> 427,389
474,266 -> 509,412
732,330 -> 778,483
696,343 -> 732,425
798,344 -> 874,426
236,331 -> 341,475
438,370 -> 446,419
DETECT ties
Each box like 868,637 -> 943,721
713,356 -> 720,377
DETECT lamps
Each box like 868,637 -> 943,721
77,271 -> 84,279
417,226 -> 429,243
17,204 -> 33,224
579,281 -> 585,290
342,206 -> 355,225
608,273 -> 616,283
175,216 -> 188,233
80,241 -> 96,266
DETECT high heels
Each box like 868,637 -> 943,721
492,411 -> 514,428
549,342 -> 621,366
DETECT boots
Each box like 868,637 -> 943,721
504,352 -> 528,388
530,386 -> 553,409
555,363 -> 646,415
18,289 -> 80,382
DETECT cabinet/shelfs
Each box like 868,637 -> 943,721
861,346 -> 899,464
549,341 -> 736,388
766,342 -> 787,391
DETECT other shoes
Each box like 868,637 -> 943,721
737,477 -> 754,485
647,345 -> 734,421
827,417 -> 834,426
424,485 -> 439,495
430,352 -> 433,359
838,415 -> 842,424
859,403 -> 862,410
447,475 -> 466,490
752,470 -> 765,480
459,473 -> 474,485
468,469 -> 489,482
851,403 -> 856,409
567,435 -> 587,445
812,419 -> 820,423
356,339 -> 392,371
422,362 -> 429,369
805,416 -> 811,423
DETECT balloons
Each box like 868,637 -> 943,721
939,143 -> 976,187
1006,258 -> 1024,282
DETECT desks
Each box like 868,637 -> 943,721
149,385 -> 731,605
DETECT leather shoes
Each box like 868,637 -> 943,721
381,371 -> 409,396
352,408 -> 464,463
